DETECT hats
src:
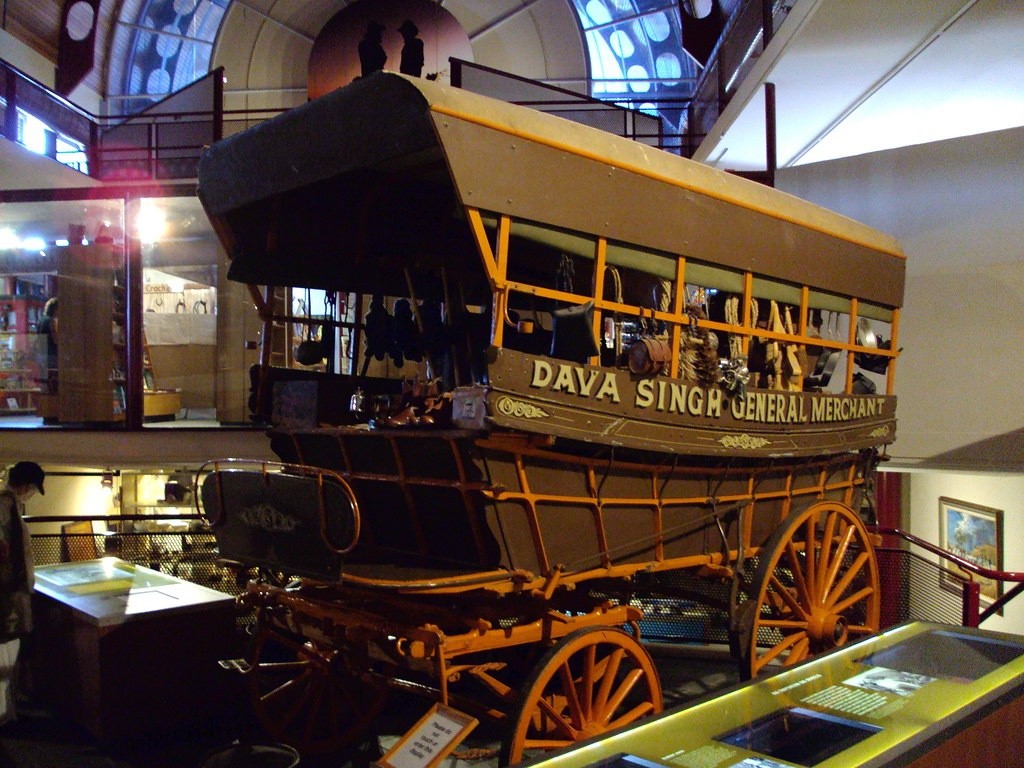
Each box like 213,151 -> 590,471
7,461 -> 46,497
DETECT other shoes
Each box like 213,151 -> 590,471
2,712 -> 39,741
411,391 -> 452,427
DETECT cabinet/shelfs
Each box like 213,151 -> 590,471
0,226 -> 180,425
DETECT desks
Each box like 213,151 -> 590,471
31,556 -> 237,748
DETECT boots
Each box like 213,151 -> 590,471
375,374 -> 442,428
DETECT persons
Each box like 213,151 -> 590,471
357,21 -> 387,77
397,20 -> 425,78
40,297 -> 59,426
0,462 -> 46,735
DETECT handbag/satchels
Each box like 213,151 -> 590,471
629,335 -> 672,377
550,304 -> 599,359
